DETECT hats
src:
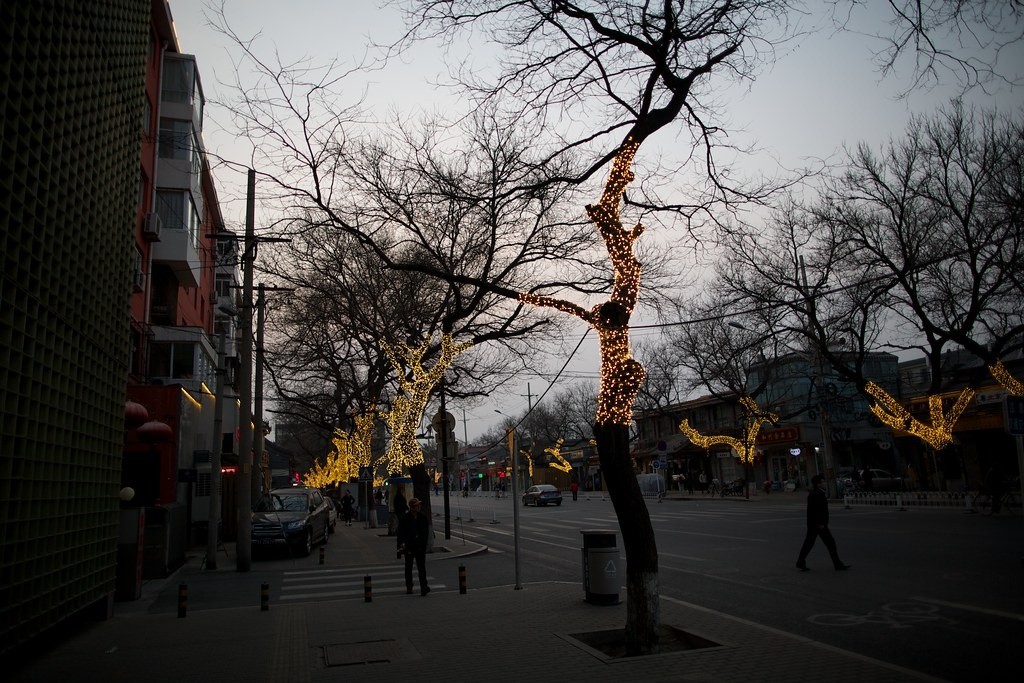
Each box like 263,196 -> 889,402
409,498 -> 422,506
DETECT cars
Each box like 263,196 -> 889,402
522,485 -> 562,507
323,496 -> 337,534
836,468 -> 902,492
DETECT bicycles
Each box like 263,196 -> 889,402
711,481 -> 743,497
974,477 -> 1024,516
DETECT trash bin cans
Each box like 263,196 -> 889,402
580,528 -> 623,606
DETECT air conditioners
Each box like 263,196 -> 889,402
211,291 -> 218,303
144,212 -> 162,242
135,268 -> 146,291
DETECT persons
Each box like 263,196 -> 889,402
711,475 -> 720,497
500,482 -> 506,498
851,465 -> 862,482
904,464 -> 920,492
493,481 -> 501,498
569,480 -> 579,501
595,477 -> 601,491
862,464 -> 873,491
376,489 -> 383,504
463,484 -> 468,498
699,471 -> 708,494
983,463 -> 1008,514
795,475 -> 851,572
340,489 -> 355,526
397,499 -> 430,597
836,470 -> 849,499
382,486 -> 389,504
434,485 -> 438,495
678,471 -> 694,495
394,487 -> 409,536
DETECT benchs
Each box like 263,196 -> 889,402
726,479 -> 743,496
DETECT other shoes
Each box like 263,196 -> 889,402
836,565 -> 850,570
407,587 -> 413,594
421,587 -> 429,596
796,565 -> 811,571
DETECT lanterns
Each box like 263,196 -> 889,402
487,469 -> 494,477
124,401 -> 149,444
497,470 -> 506,478
136,419 -> 173,448
293,471 -> 300,484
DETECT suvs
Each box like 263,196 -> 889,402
252,488 -> 329,556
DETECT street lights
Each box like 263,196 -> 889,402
218,304 -> 252,572
494,410 -> 534,485
728,321 -> 838,499
